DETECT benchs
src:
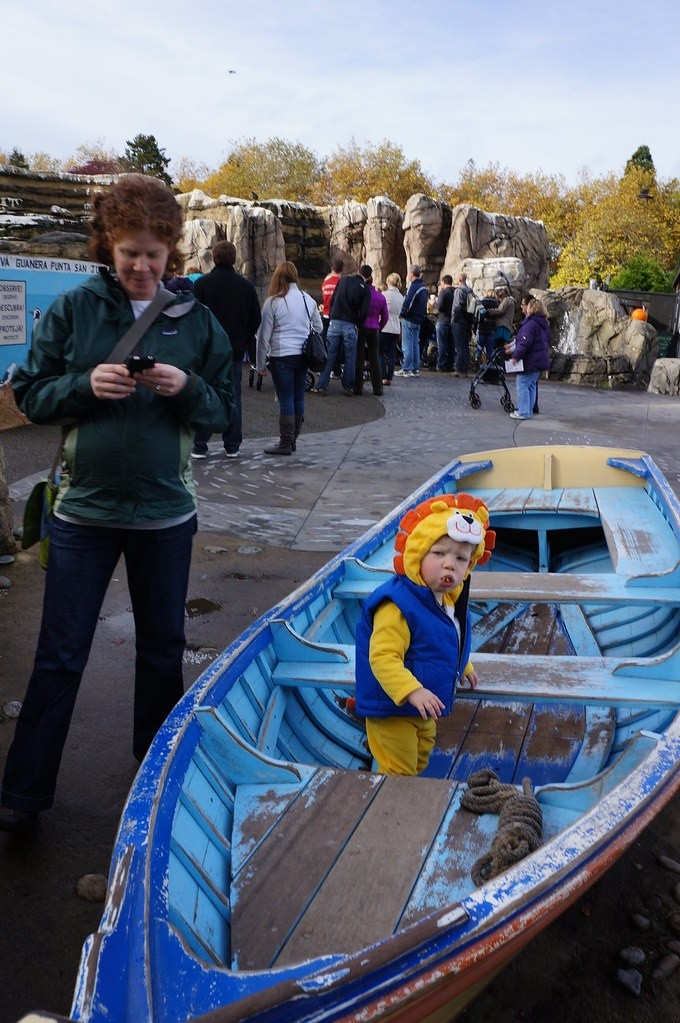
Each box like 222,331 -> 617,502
268,558 -> 679,708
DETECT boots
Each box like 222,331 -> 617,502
264,414 -> 304,455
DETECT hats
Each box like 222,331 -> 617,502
395,493 -> 496,604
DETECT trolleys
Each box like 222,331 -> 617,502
249,353 -> 317,392
468,338 -> 516,413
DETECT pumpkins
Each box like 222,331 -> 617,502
632,304 -> 647,321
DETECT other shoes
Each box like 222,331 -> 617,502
227,451 -> 239,457
310,386 -> 326,397
0,802 -> 37,831
344,388 -> 353,396
450,371 -> 467,378
382,378 -> 392,386
191,450 -> 207,459
510,408 -> 531,420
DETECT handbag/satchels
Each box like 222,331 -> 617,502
20,477 -> 55,572
300,328 -> 330,372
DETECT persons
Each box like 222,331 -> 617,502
381,272 -> 404,385
311,265 -> 371,395
393,264 -> 428,377
428,273 -> 456,372
304,259 -> 343,380
586,268 -> 608,292
257,262 -> 324,455
168,241 -> 263,460
472,285 -> 552,420
354,276 -> 389,395
448,271 -> 473,377
355,493 -> 496,777
0,178 -> 237,833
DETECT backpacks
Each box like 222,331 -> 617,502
458,286 -> 485,324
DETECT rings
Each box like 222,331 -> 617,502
154,385 -> 160,393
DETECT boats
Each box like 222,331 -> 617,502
67,445 -> 680,1022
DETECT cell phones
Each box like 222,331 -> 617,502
126,356 -> 153,378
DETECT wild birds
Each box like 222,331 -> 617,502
228,69 -> 236,74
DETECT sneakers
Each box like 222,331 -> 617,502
394,369 -> 419,378
